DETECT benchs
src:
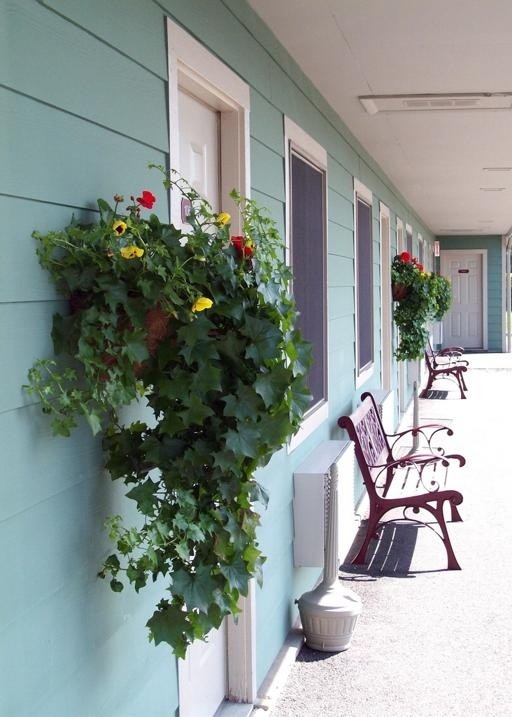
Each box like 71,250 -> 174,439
420,335 -> 470,400
337,390 -> 467,572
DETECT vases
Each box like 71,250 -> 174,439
74,298 -> 175,387
393,283 -> 410,301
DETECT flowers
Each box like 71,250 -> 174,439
21,188 -> 308,665
392,252 -> 452,363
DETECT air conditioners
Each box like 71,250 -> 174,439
370,390 -> 395,435
294,439 -> 357,568
